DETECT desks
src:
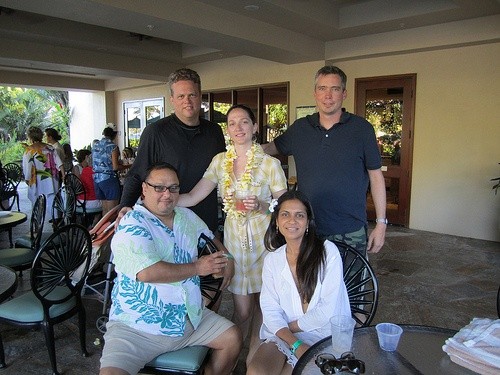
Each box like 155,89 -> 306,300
291,324 -> 500,375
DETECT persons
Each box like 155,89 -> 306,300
7,134 -> 11,142
23,126 -> 65,234
20,129 -> 65,227
175,103 -> 288,371
244,191 -> 352,375
99,163 -> 243,375
114,68 -> 228,267
63,120 -> 137,233
259,64 -> 387,319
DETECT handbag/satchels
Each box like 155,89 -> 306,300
65,204 -> 124,296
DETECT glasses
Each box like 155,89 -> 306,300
146,181 -> 181,193
314,351 -> 355,369
320,358 -> 365,375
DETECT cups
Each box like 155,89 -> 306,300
235,189 -> 251,212
210,254 -> 228,279
375,322 -> 403,352
329,315 -> 356,352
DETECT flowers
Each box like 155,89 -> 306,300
265,199 -> 278,214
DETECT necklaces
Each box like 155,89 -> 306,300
221,141 -> 265,218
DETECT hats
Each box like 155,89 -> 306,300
105,122 -> 118,132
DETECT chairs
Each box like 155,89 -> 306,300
0,162 -> 377,375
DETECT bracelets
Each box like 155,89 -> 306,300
266,203 -> 273,216
376,218 -> 389,226
289,339 -> 302,355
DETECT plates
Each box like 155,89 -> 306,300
0,211 -> 11,217
